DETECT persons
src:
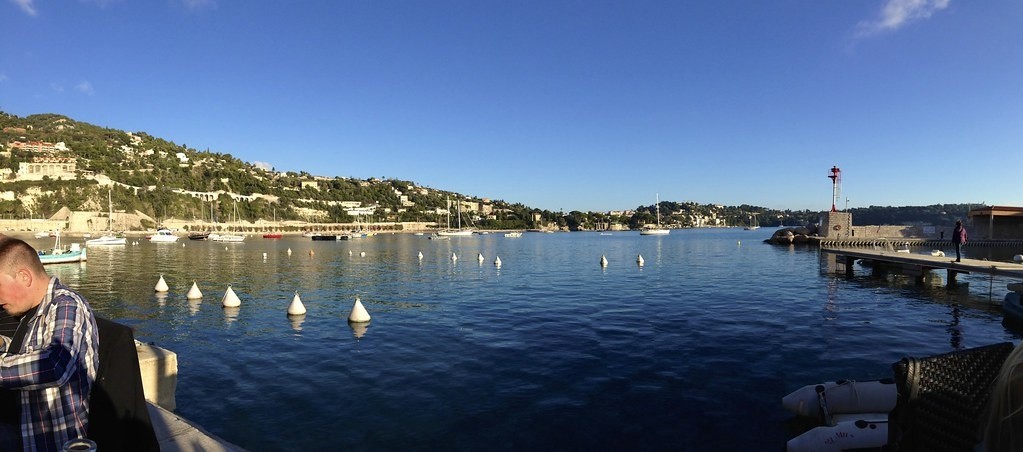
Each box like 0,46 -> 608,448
952,220 -> 967,261
0,239 -> 99,452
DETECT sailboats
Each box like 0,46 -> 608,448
639,192 -> 670,236
301,203 -> 378,241
413,216 -> 423,236
437,195 -> 473,235
144,194 -> 248,243
262,208 -> 283,239
84,189 -> 128,246
744,215 -> 761,230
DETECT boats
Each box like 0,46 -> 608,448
504,230 -> 522,238
782,378 -> 898,452
36,233 -> 88,265
478,227 -> 489,235
427,231 -> 448,240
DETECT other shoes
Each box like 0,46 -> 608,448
956,259 -> 960,262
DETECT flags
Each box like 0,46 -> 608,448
877,225 -> 881,233
